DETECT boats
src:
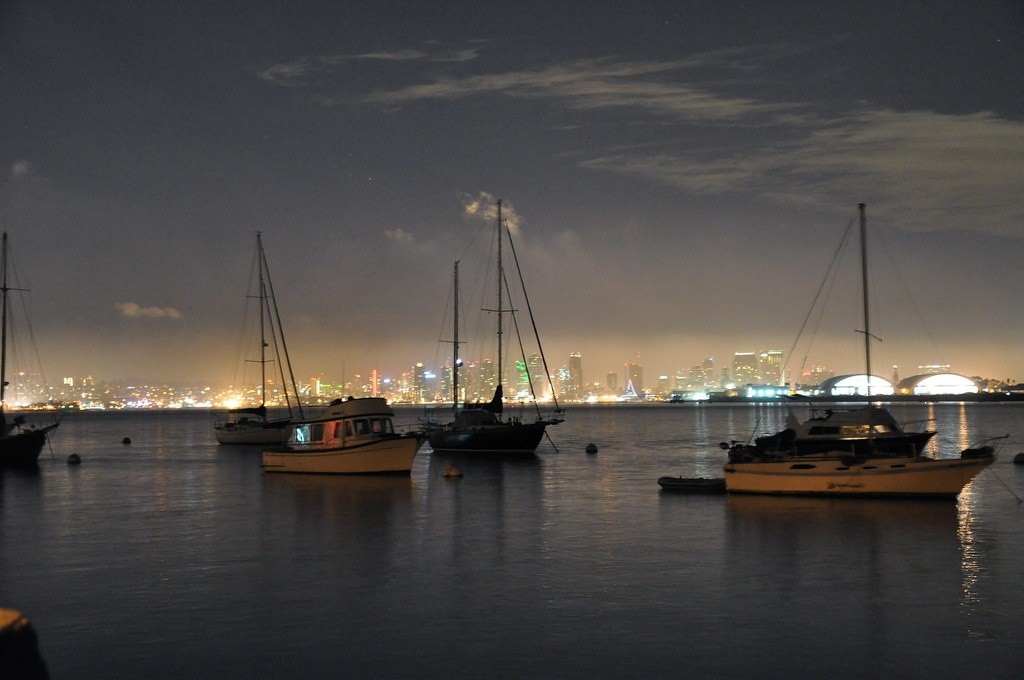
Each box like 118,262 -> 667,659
260,395 -> 429,475
658,475 -> 726,491
1,229 -> 61,469
725,202 -> 1001,500
214,231 -> 323,452
424,197 -> 565,459
